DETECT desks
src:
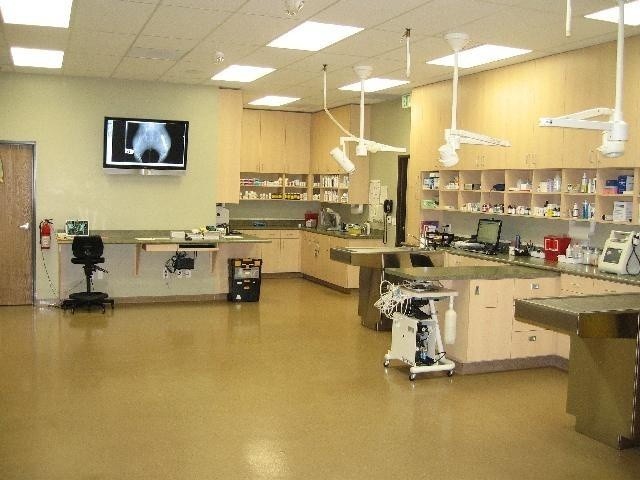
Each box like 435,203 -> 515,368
56,227 -> 273,309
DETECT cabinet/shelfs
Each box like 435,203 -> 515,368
221,170 -> 639,375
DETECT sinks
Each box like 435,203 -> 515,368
397,247 -> 430,251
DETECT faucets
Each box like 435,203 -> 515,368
407,234 -> 430,250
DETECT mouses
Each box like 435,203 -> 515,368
486,250 -> 496,255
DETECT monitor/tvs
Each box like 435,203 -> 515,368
476,219 -> 502,249
103,117 -> 189,176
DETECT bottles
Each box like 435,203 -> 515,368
482,203 -> 530,215
544,200 -> 560,217
285,193 -> 300,200
566,244 -> 600,266
572,201 -> 591,219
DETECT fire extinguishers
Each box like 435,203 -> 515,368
39,218 -> 53,249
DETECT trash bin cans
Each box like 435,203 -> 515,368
227,258 -> 262,301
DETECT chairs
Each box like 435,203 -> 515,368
62,234 -> 116,315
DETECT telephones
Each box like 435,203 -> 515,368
384,200 -> 393,213
